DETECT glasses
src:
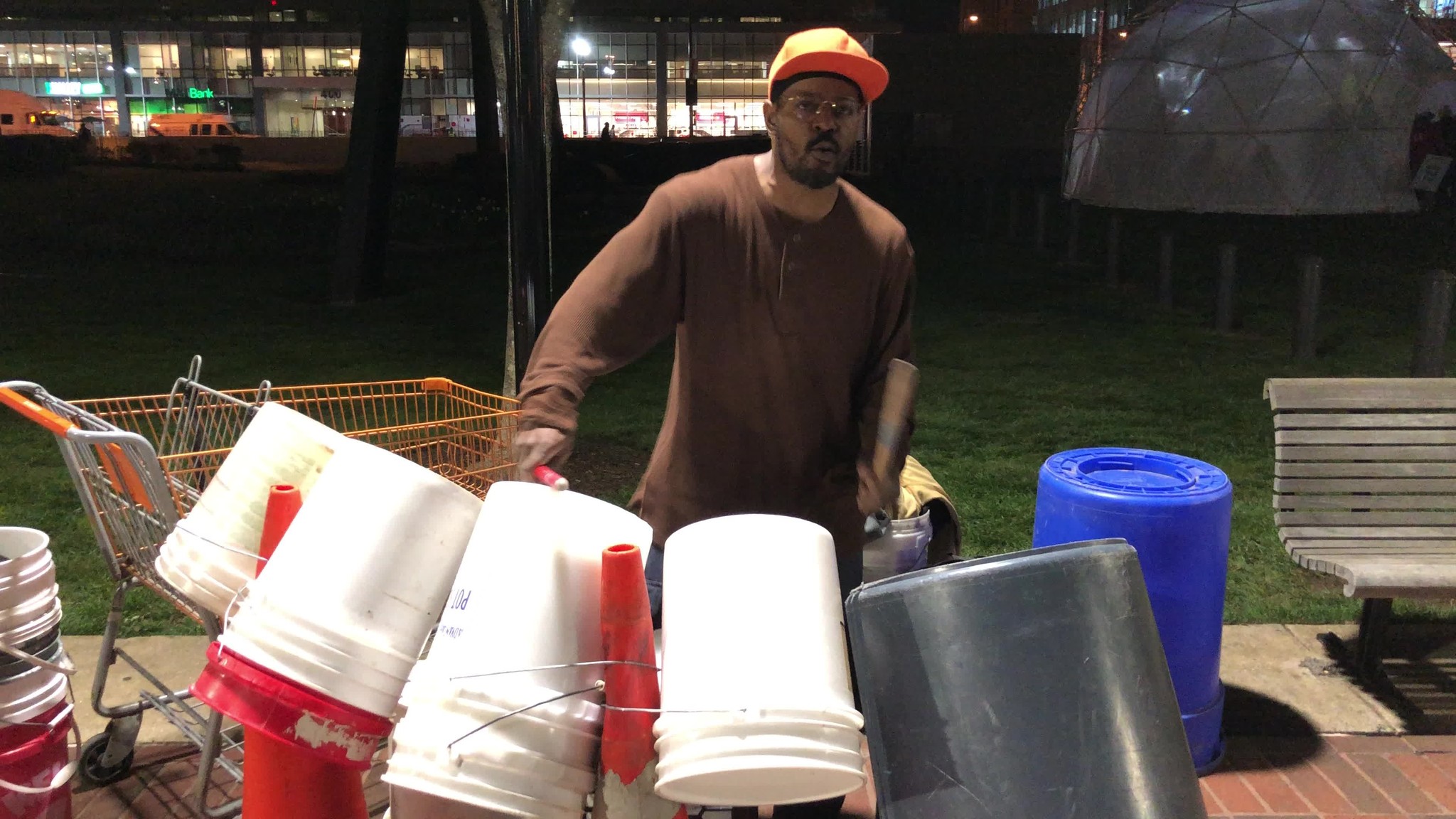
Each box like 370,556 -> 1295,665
784,96 -> 865,123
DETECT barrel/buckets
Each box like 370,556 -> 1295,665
845,536 -> 1208,819
154,396 -> 346,614
862,509 -> 933,586
186,438 -> 656,818
1035,446 -> 1232,774
653,512 -> 863,808
0,524 -> 81,819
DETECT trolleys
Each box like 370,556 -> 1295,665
0,353 -> 523,819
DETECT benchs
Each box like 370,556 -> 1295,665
1262,377 -> 1456,724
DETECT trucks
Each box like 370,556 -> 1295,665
149,113 -> 256,137
1,90 -> 82,138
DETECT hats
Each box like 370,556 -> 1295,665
768,27 -> 890,102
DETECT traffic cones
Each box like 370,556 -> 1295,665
242,482 -> 368,819
596,541 -> 688,819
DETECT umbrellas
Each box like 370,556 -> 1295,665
44,113 -> 106,122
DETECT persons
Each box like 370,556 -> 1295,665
483,23 -> 924,819
611,125 -> 615,137
601,122 -> 610,138
78,124 -> 92,147
